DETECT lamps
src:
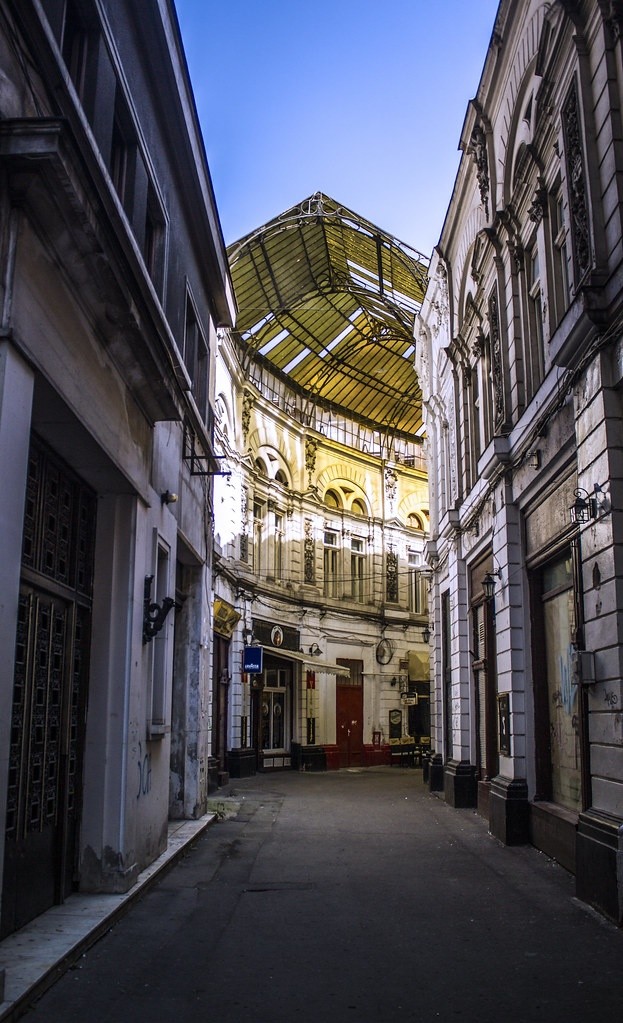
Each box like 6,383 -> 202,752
568,488 -> 597,523
309,642 -> 323,657
481,566 -> 501,597
421,622 -> 435,644
